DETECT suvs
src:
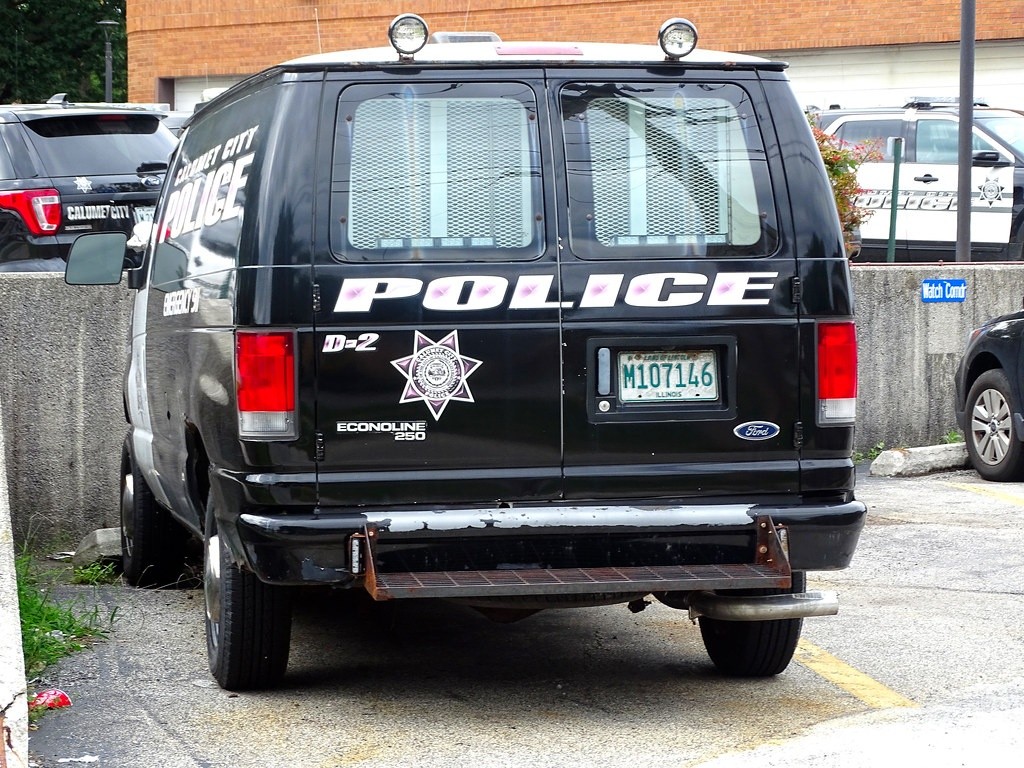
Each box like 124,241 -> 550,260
739,97 -> 1023,260
0,94 -> 179,272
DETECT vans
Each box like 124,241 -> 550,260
62,15 -> 866,695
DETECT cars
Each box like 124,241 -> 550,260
955,309 -> 1024,483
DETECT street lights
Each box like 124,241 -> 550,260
96,19 -> 119,103
